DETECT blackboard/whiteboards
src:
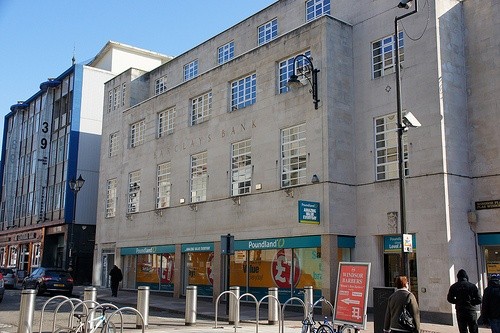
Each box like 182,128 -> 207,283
17,269 -> 25,282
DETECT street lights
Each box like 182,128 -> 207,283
68,174 -> 85,276
394,0 -> 419,290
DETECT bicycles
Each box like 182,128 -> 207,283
51,304 -> 116,333
301,310 -> 357,333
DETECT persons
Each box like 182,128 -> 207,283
67,258 -> 75,275
481,281 -> 500,333
383,275 -> 420,333
109,265 -> 123,297
447,269 -> 482,333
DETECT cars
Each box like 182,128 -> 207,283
22,267 -> 74,296
0,268 -> 16,289
0,267 -> 4,303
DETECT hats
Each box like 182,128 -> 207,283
456,269 -> 467,278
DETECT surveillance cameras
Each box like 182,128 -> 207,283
401,111 -> 421,132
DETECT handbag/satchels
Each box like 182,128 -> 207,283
477,310 -> 490,328
398,292 -> 417,332
